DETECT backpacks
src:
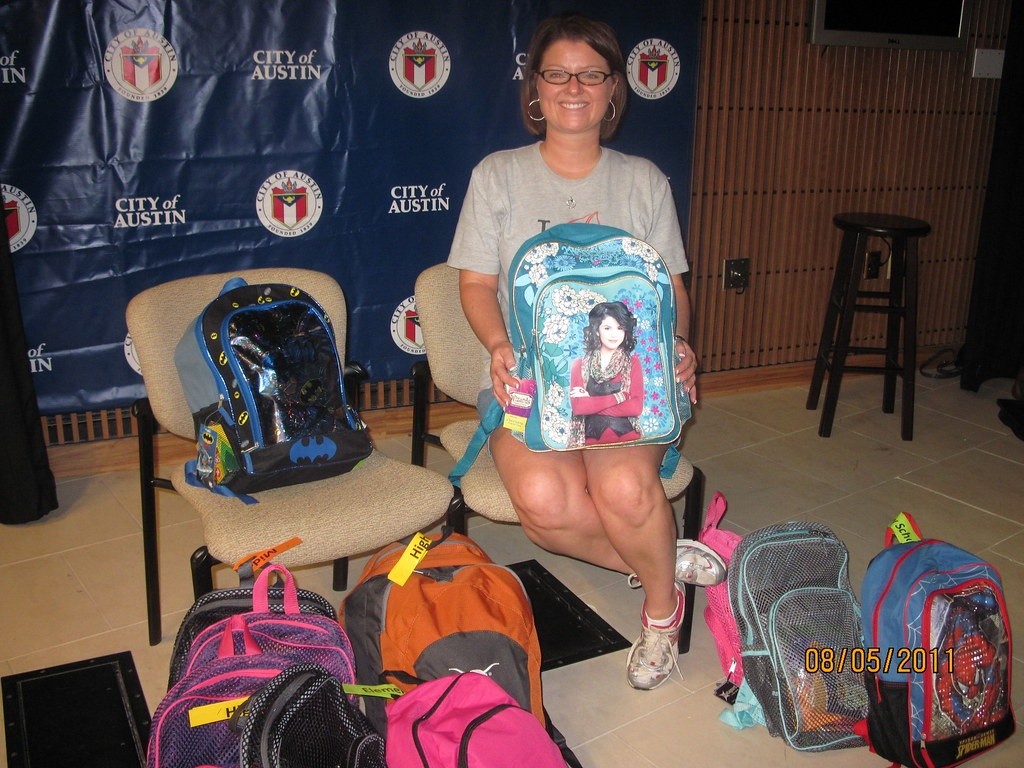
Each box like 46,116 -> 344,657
698,490 -> 746,689
853,512 -> 1016,768
483,223 -> 693,452
146,533 -> 582,768
174,277 -> 373,496
719,522 -> 868,752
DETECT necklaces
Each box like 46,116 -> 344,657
564,190 -> 577,209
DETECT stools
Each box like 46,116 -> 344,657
805,212 -> 932,444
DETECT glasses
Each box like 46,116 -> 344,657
536,69 -> 616,85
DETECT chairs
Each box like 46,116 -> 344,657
411,262 -> 705,655
125,267 -> 467,647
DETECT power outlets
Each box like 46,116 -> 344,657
863,251 -> 881,279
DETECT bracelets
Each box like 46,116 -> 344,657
676,335 -> 686,342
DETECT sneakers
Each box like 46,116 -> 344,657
627,539 -> 726,588
625,579 -> 686,691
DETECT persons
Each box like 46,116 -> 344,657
446,21 -> 728,691
571,301 -> 645,445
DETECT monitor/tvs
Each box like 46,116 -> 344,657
808,0 -> 972,53
